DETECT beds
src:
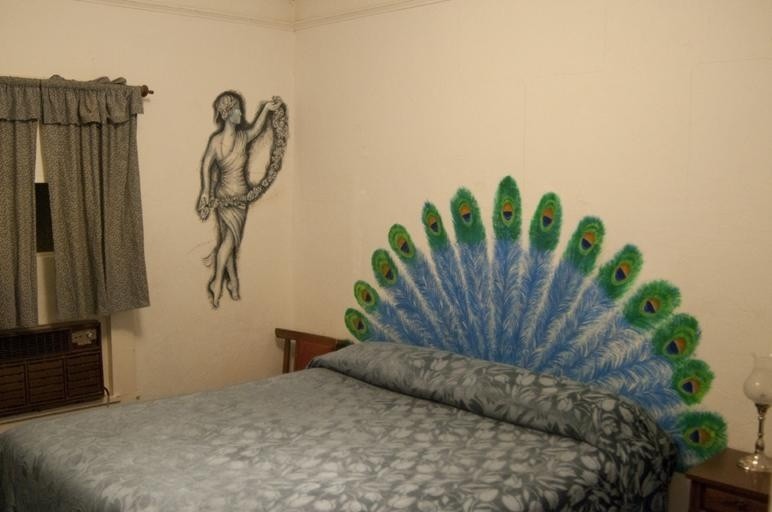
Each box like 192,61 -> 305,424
0,327 -> 728,511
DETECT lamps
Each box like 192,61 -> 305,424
738,353 -> 771,473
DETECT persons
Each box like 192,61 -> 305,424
197,91 -> 281,307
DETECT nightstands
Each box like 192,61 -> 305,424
685,445 -> 772,512
